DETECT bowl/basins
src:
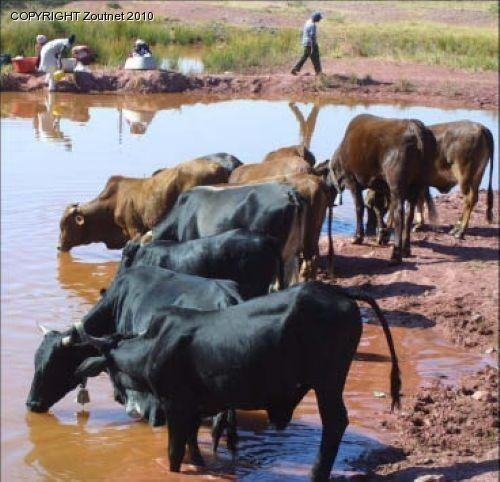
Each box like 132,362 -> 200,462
125,56 -> 156,70
10,103 -> 37,119
11,56 -> 38,73
124,109 -> 153,123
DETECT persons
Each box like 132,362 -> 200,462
38,34 -> 76,91
290,10 -> 324,77
288,101 -> 321,150
128,39 -> 152,58
130,110 -> 156,136
38,91 -> 72,152
34,35 -> 49,74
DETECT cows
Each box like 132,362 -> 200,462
81,280 -> 402,478
24,264 -> 247,464
314,114 -> 436,267
364,120 -> 493,239
56,143 -> 336,301
